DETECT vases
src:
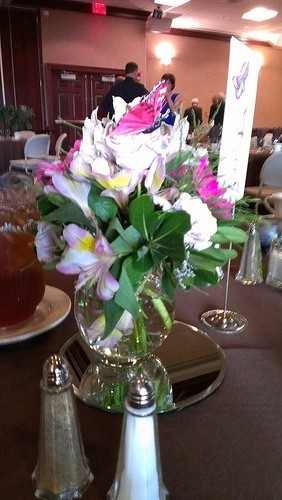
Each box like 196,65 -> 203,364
74,273 -> 176,414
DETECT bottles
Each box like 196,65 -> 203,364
266,236 -> 282,290
235,219 -> 263,285
104,373 -> 170,500
31,354 -> 95,500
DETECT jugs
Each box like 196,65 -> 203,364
0,171 -> 46,327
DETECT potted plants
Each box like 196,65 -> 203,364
0,104 -> 36,141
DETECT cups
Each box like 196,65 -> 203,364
263,192 -> 282,219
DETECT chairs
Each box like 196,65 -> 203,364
14,130 -> 35,140
25,133 -> 66,175
246,151 -> 282,216
9,134 -> 51,173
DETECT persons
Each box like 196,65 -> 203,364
159,73 -> 182,127
101,62 -> 149,122
183,98 -> 203,145
208,92 -> 225,127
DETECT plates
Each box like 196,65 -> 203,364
0,284 -> 72,345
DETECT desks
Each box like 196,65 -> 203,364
0,252 -> 282,500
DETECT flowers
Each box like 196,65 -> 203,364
32,79 -> 270,411
